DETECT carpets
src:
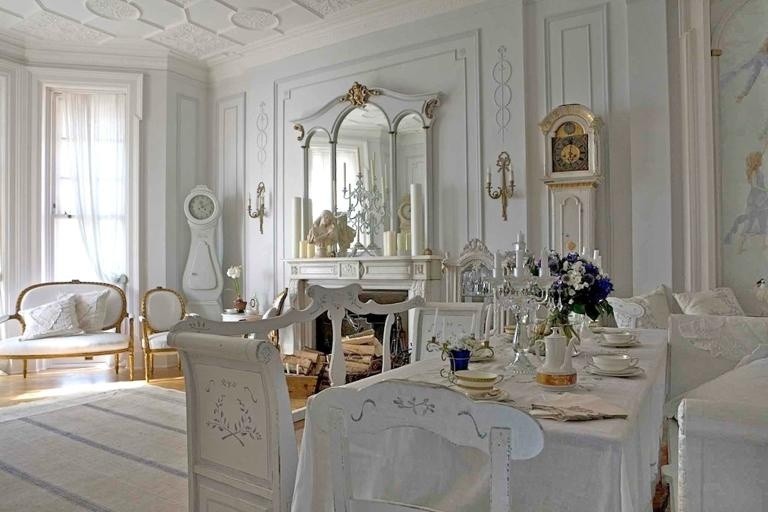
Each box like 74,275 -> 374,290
0,381 -> 188,512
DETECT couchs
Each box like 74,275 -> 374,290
668,313 -> 768,511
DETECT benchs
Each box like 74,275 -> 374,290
0,280 -> 134,380
171,284 -> 426,459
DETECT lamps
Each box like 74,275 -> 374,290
248,182 -> 266,234
484,152 -> 515,221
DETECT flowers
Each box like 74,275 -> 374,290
523,249 -> 613,357
226,265 -> 242,297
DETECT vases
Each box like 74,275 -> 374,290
232,296 -> 247,313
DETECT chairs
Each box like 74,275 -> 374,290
140,286 -> 185,382
411,303 -> 483,363
167,332 -> 297,511
290,380 -> 545,511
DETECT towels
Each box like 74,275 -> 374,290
529,392 -> 627,422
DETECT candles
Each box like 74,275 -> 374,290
358,148 -> 361,172
516,231 -> 525,243
373,152 -> 375,160
541,247 -> 548,267
385,164 -> 388,188
381,177 -> 385,204
370,160 -> 375,187
344,163 -> 346,187
494,248 -> 501,267
349,184 -> 351,207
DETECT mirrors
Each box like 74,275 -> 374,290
290,80 -> 443,257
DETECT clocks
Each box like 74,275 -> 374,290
184,191 -> 218,225
398,201 -> 411,222
552,121 -> 589,171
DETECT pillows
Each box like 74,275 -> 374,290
606,285 -> 747,328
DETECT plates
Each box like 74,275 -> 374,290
583,365 -> 644,379
594,341 -> 642,348
466,386 -> 509,404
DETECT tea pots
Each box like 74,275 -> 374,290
534,326 -> 579,394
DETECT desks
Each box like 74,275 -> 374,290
220,313 -> 263,340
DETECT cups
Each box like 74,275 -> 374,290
504,325 -> 515,341
246,307 -> 257,314
601,332 -> 635,344
590,352 -> 632,373
445,349 -> 470,372
453,370 -> 506,396
225,308 -> 238,314
